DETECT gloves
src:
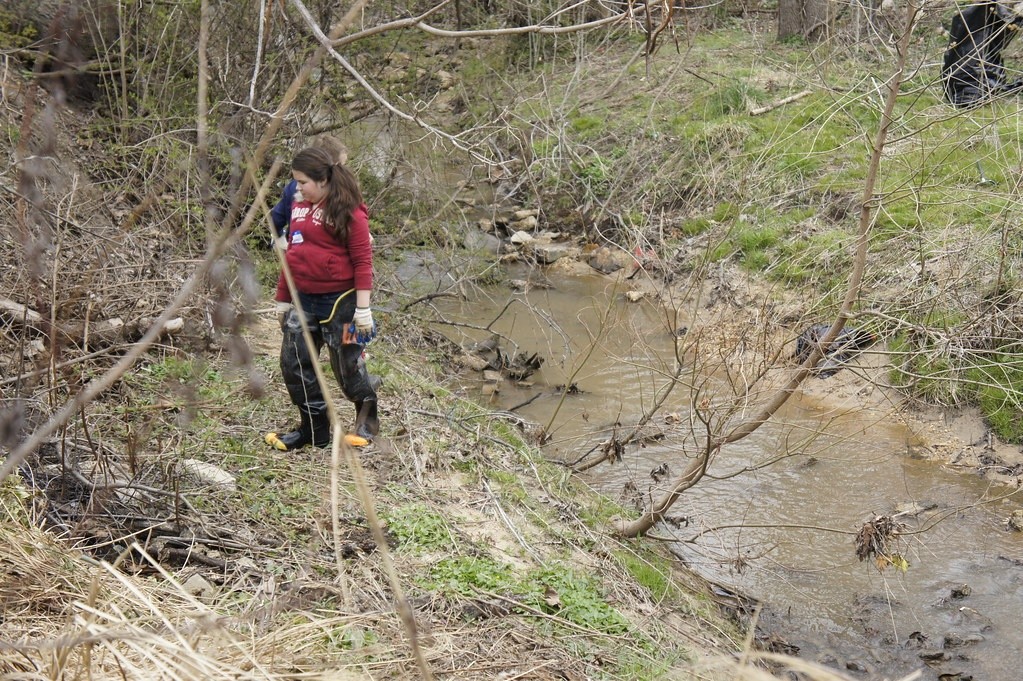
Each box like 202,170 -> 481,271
348,307 -> 376,343
277,302 -> 292,326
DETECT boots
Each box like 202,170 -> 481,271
322,320 -> 379,446
265,306 -> 330,451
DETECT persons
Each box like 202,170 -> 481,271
261,148 -> 381,451
262,136 -> 348,251
